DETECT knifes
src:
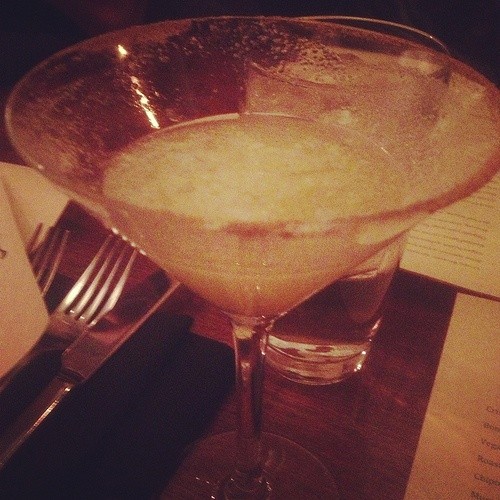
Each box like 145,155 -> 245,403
0,277 -> 181,469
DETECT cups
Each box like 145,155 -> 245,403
247,15 -> 450,387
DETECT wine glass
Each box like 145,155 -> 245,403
5,17 -> 500,500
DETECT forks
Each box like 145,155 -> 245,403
0,235 -> 137,394
26,224 -> 69,299
